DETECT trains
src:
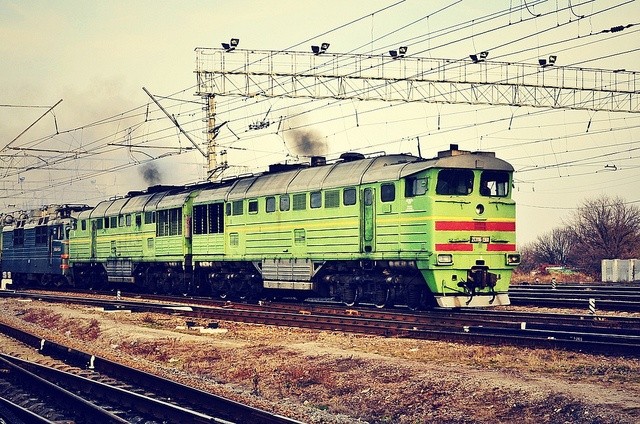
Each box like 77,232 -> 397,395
0,145 -> 521,309
